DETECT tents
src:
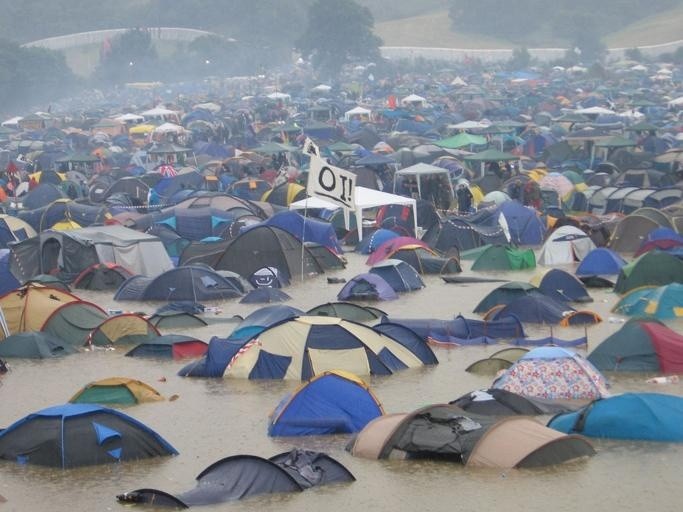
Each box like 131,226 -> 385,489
0,60 -> 682,511
